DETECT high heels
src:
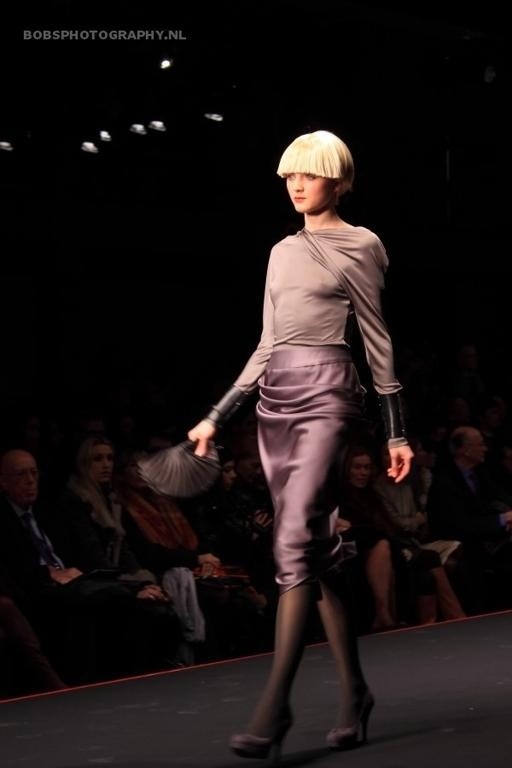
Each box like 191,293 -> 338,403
325,691 -> 376,748
229,709 -> 294,759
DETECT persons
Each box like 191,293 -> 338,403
186,130 -> 415,760
